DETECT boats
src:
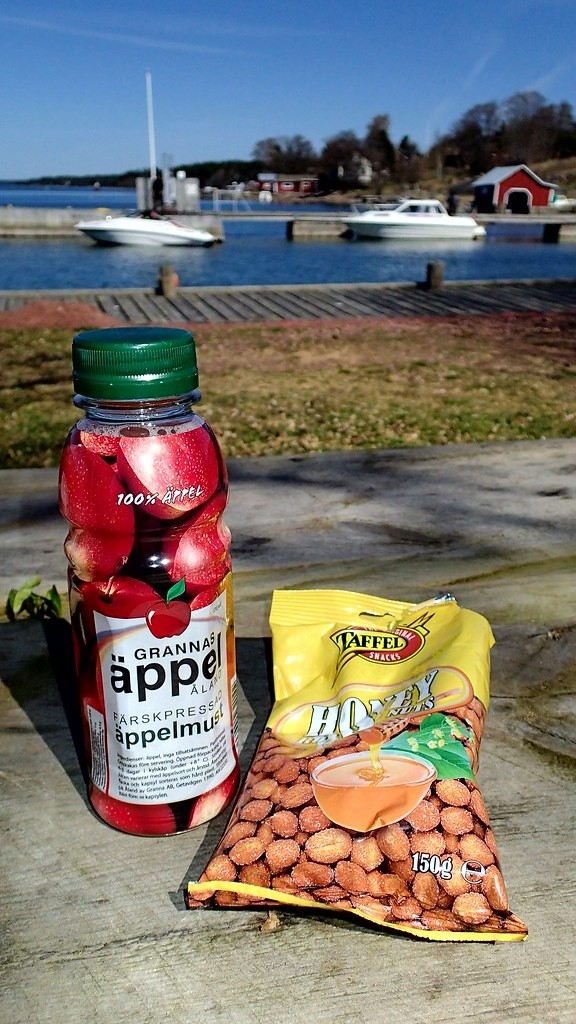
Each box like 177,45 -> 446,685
341,198 -> 488,241
74,205 -> 225,250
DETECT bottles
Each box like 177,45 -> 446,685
58,328 -> 242,838
